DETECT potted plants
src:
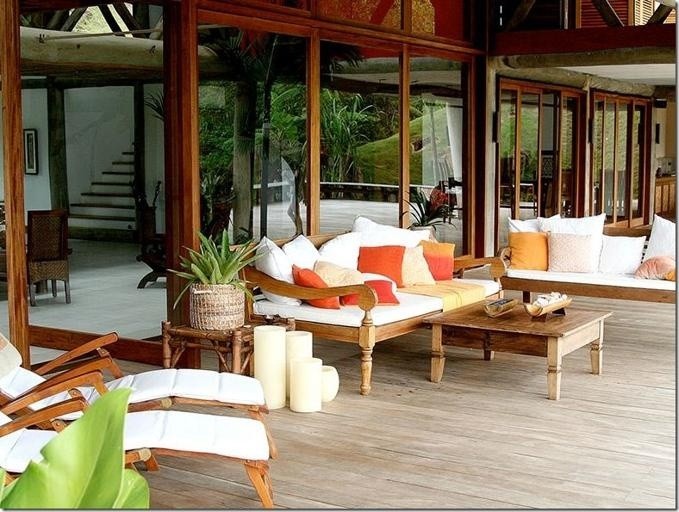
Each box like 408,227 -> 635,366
166,228 -> 273,333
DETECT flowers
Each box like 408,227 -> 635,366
399,189 -> 462,230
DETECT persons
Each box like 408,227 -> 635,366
285,159 -> 306,238
427,181 -> 450,216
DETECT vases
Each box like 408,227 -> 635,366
250,322 -> 339,415
410,223 -> 435,234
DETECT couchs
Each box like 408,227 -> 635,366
491,220 -> 676,321
235,228 -> 508,396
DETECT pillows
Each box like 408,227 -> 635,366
633,252 -> 676,282
504,230 -> 550,272
535,212 -> 607,232
544,227 -> 604,273
597,234 -> 648,275
251,215 -> 458,311
505,212 -> 562,233
644,211 -> 676,258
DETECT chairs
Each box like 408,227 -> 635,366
0,331 -> 279,461
24,206 -> 71,308
135,197 -> 167,290
0,368 -> 281,511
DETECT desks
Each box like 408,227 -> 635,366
160,317 -> 298,383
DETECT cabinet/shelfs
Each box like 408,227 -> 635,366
654,180 -> 676,217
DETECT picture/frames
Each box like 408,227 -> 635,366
22,126 -> 41,175
